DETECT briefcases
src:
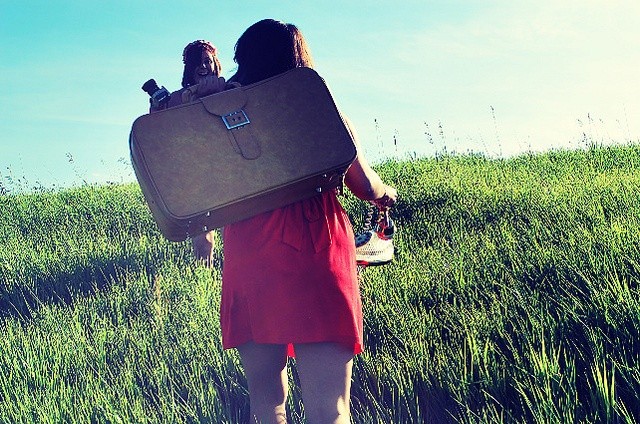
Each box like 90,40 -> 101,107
128,66 -> 360,243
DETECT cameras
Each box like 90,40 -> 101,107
141,79 -> 172,106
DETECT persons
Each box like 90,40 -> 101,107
144,39 -> 226,267
209,20 -> 396,424
